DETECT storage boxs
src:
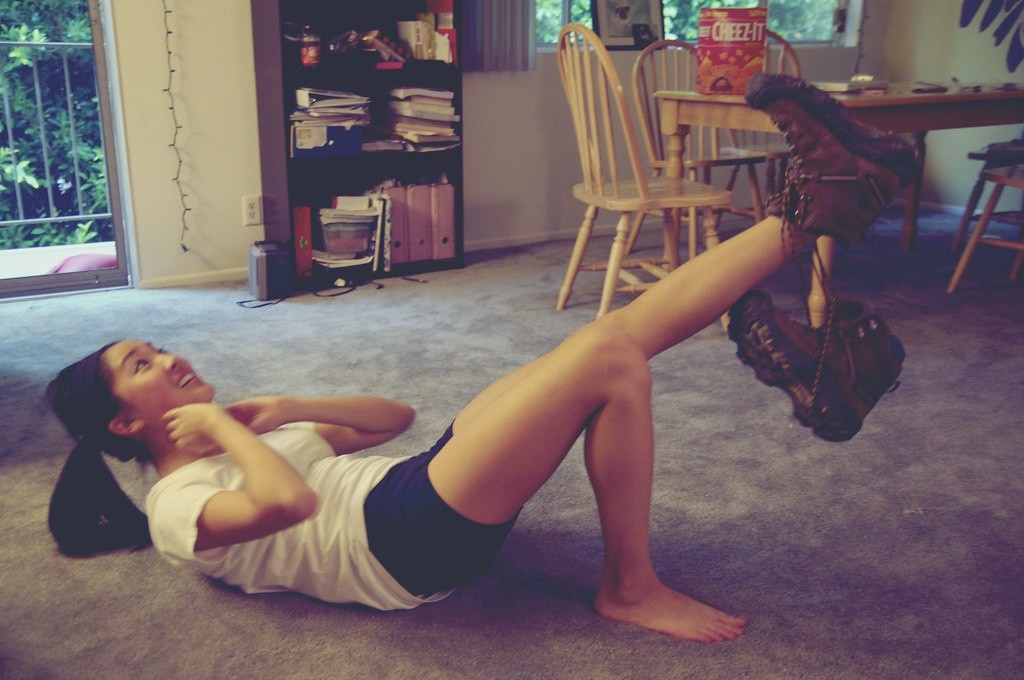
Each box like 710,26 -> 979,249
287,119 -> 363,159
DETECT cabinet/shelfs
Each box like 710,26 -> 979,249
251,0 -> 464,296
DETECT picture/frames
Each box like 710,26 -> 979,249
590,0 -> 665,50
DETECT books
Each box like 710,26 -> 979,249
312,250 -> 374,268
289,87 -> 369,128
397,13 -> 435,60
389,86 -> 460,144
319,195 -> 378,225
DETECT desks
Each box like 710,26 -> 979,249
651,82 -> 1024,275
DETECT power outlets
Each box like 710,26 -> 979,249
243,195 -> 261,226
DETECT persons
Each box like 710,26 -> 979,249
46,72 -> 918,645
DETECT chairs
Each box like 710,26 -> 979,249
714,29 -> 800,234
945,164 -> 1024,293
950,139 -> 1024,257
555,24 -> 732,332
625,41 -> 766,334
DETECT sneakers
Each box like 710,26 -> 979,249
727,281 -> 906,442
745,72 -> 915,279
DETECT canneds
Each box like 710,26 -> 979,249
300,25 -> 321,66
